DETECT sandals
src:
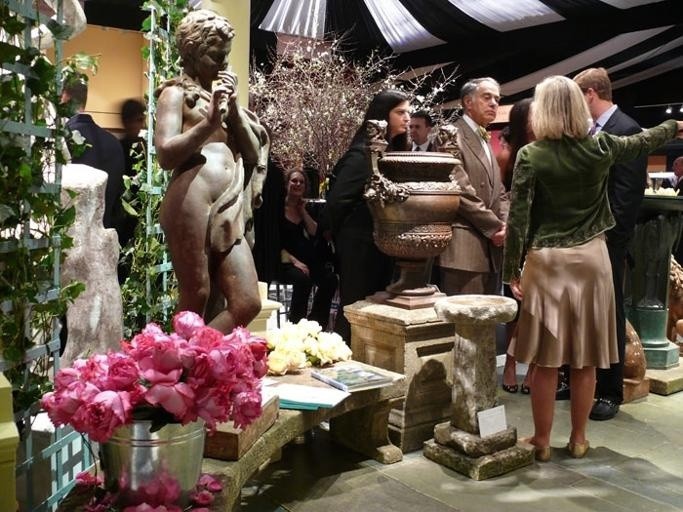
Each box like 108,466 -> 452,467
502,382 -> 518,393
521,382 -> 530,394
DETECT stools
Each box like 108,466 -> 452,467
265,281 -> 318,328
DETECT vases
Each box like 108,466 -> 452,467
98,416 -> 206,498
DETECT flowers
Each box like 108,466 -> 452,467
265,318 -> 353,376
38,311 -> 269,443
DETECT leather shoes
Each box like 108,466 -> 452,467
588,397 -> 619,420
518,436 -> 550,461
555,381 -> 572,400
568,439 -> 590,458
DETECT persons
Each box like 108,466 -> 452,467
154,8 -> 271,335
59,71 -> 126,358
266,67 -> 682,461
118,99 -> 149,286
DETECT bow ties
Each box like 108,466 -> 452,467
479,126 -> 491,143
